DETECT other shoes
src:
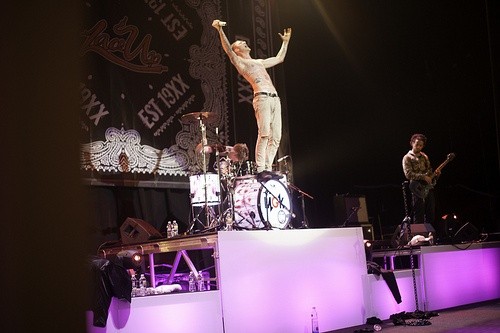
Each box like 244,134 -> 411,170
267,172 -> 283,179
257,173 -> 273,181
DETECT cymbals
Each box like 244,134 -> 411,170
273,163 -> 279,167
210,143 -> 226,152
181,111 -> 213,120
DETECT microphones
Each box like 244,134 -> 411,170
278,155 -> 289,162
351,207 -> 360,209
218,21 -> 227,26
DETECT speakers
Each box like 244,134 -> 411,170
389,223 -> 438,249
452,222 -> 481,244
120,218 -> 162,243
334,196 -> 369,224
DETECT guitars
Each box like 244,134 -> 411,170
409,152 -> 456,199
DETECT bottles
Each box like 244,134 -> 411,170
139,274 -> 147,296
131,274 -> 137,298
189,272 -> 196,292
167,220 -> 178,238
196,270 -> 204,291
310,306 -> 319,333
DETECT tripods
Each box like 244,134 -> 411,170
183,118 -> 259,236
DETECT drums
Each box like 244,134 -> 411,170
238,161 -> 257,176
226,178 -> 294,230
188,173 -> 222,207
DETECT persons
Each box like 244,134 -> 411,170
212,19 -> 292,180
402,134 -> 441,224
196,141 -> 249,212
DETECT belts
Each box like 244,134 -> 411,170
254,92 -> 278,97
